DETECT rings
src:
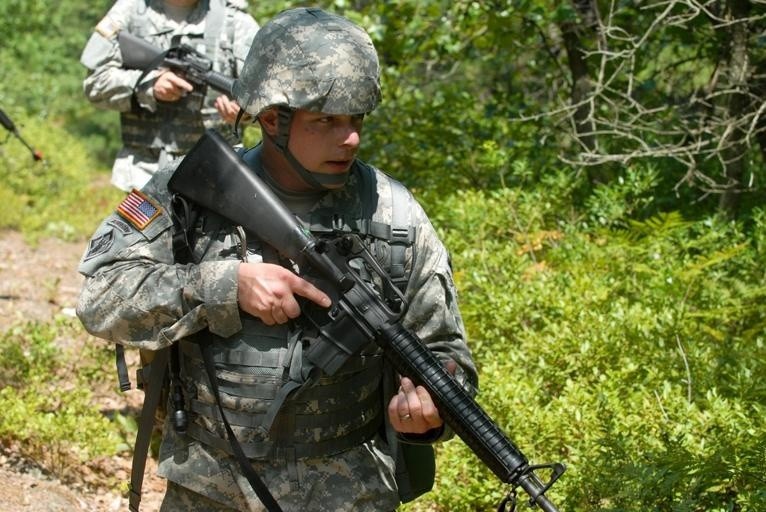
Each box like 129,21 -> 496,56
398,412 -> 414,422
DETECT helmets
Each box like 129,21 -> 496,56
231,5 -> 381,115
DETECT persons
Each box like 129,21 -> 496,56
74,5 -> 481,512
80,1 -> 261,198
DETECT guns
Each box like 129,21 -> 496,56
0,110 -> 43,160
166,130 -> 567,511
119,30 -> 235,116
